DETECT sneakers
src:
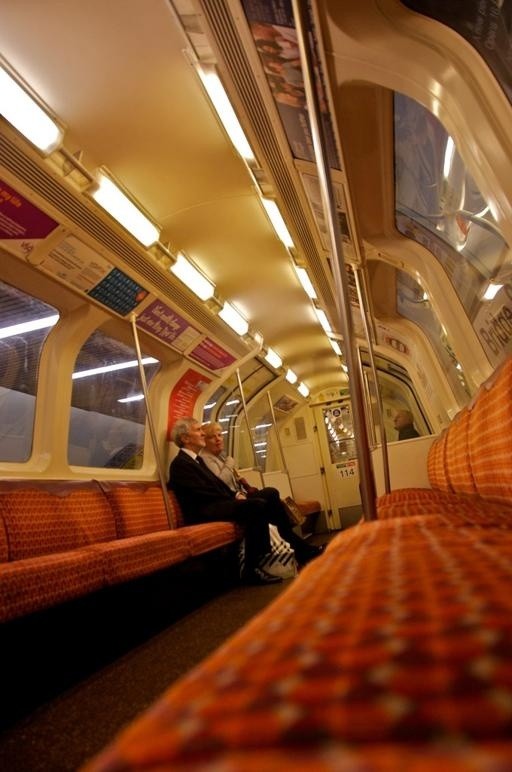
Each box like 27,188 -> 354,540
309,545 -> 324,559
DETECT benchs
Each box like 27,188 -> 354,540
0,353 -> 512,772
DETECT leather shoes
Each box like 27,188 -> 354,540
242,569 -> 282,586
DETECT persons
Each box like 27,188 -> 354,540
199,420 -> 249,496
393,409 -> 420,440
168,417 -> 321,586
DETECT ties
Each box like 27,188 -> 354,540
196,456 -> 227,492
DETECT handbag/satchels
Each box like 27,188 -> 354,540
280,496 -> 307,526
